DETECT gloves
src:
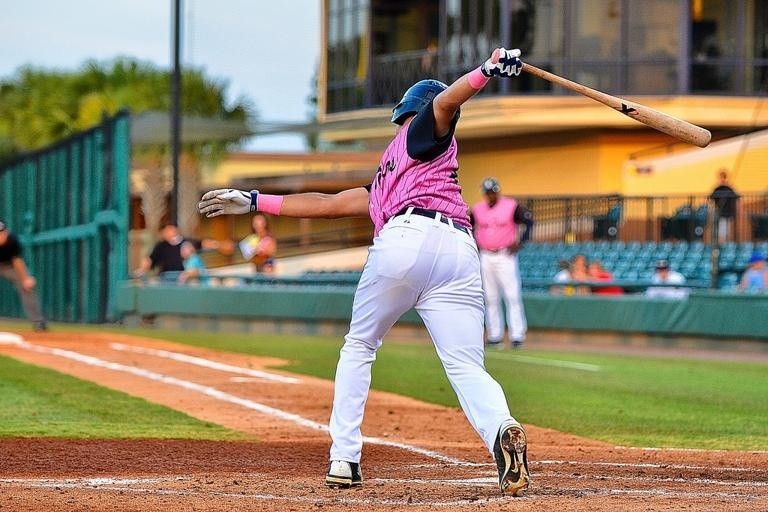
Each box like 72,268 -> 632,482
198,188 -> 259,218
482,47 -> 523,78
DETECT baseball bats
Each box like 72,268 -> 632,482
490,49 -> 712,148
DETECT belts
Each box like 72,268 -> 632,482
395,208 -> 468,233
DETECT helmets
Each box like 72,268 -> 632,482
390,79 -> 460,126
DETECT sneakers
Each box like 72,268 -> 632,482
326,461 -> 363,485
493,420 -> 529,497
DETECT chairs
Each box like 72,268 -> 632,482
517,238 -> 767,296
240,267 -> 362,296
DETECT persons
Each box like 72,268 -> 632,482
198,47 -> 530,498
470,175 -> 534,347
132,222 -> 233,285
547,170 -> 766,299
178,240 -> 211,288
0,219 -> 47,333
240,212 -> 278,274
